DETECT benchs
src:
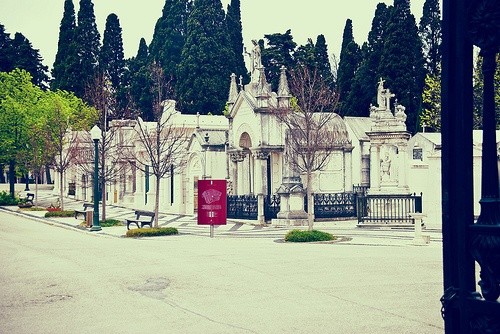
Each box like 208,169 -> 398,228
74,203 -> 94,221
19,193 -> 34,204
124,211 -> 155,230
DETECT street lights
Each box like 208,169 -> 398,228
90,125 -> 104,232
